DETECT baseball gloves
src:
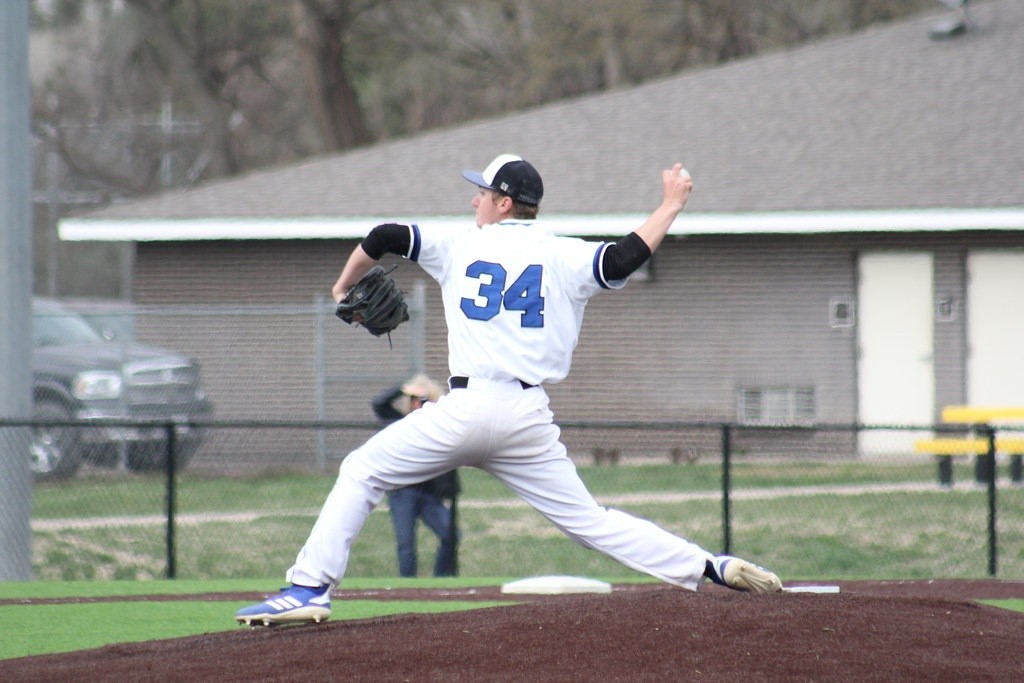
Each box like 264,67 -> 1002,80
336,265 -> 410,338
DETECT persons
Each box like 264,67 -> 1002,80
370,375 -> 464,581
233,153 -> 785,628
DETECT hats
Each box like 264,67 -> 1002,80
462,152 -> 543,205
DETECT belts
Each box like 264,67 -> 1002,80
450,375 -> 542,389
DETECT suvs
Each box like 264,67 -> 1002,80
24,297 -> 205,476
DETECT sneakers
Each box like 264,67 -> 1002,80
711,554 -> 784,596
234,588 -> 332,626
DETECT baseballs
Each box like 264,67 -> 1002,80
669,168 -> 690,178
